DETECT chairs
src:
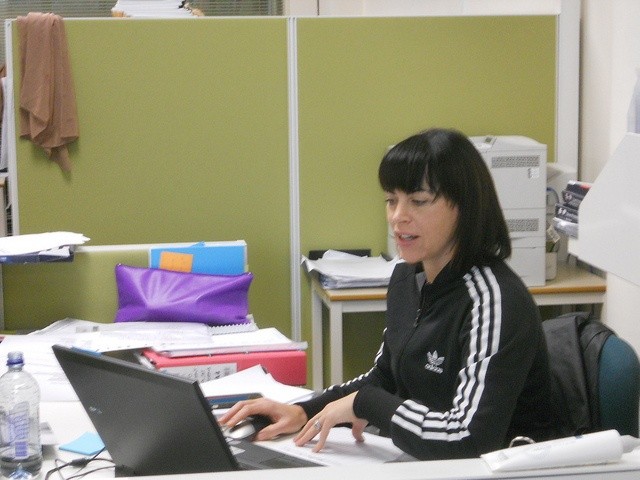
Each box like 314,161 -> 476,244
525,314 -> 640,445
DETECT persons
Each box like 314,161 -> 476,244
218,128 -> 551,459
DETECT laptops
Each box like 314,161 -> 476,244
52,345 -> 331,479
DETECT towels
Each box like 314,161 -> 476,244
16,11 -> 80,173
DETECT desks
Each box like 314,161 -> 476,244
306,261 -> 606,396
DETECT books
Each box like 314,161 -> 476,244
135,347 -> 307,390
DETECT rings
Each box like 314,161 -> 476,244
313,419 -> 321,430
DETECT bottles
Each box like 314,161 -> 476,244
0,352 -> 43,480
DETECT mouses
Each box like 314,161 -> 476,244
223,416 -> 275,441
